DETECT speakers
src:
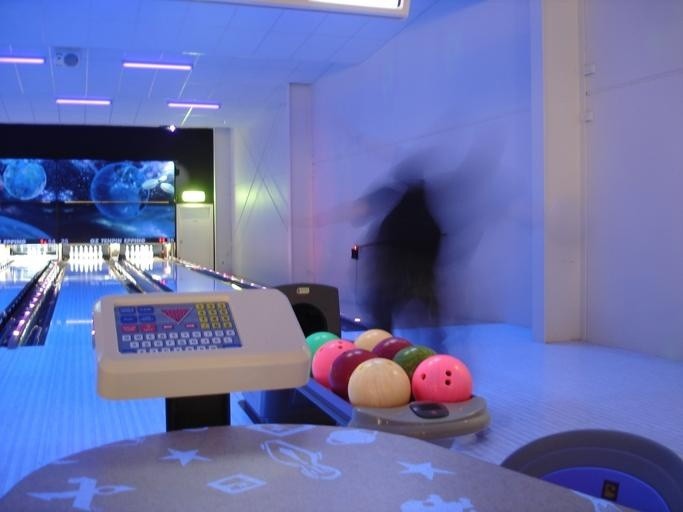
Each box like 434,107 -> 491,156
50,46 -> 87,96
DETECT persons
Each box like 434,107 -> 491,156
348,175 -> 447,358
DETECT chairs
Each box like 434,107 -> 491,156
500,428 -> 682,510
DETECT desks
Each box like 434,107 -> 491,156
0,423 -> 644,512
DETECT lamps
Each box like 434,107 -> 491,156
120,60 -> 191,72
0,55 -> 46,65
166,101 -> 222,110
53,98 -> 113,107
